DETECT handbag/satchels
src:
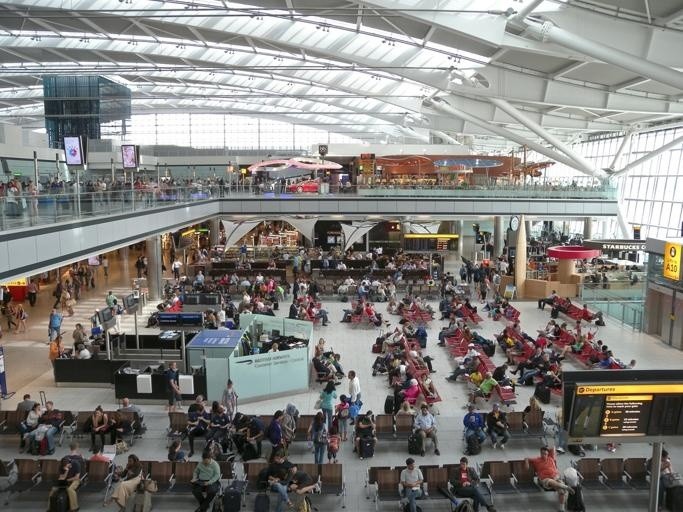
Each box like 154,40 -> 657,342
31,439 -> 47,456
565,468 -> 579,487
138,477 -> 158,493
213,493 -> 223,512
315,431 -> 328,444
125,495 -> 151,511
663,472 -> 681,487
568,445 -> 585,458
567,484 -> 585,512
298,497 -> 311,512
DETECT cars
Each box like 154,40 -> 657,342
284,177 -> 321,193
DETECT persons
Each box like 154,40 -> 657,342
658,451 -> 674,512
1,169 -> 639,511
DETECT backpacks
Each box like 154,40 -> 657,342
469,434 -> 481,454
338,405 -> 349,419
458,501 -> 474,512
328,436 -> 338,452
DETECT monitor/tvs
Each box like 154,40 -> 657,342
271,329 -> 280,337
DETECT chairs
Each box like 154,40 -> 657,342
53,246 -> 643,404
475,458 -> 560,507
569,457 -> 654,502
360,168 -> 600,197
363,464 -> 483,511
1,410 -> 147,454
463,409 -> 559,449
1,458 -> 347,509
351,413 -> 436,452
166,412 -> 322,457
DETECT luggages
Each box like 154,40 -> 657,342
666,485 -> 683,512
50,488 -> 69,511
254,481 -> 270,511
223,485 -> 240,512
360,436 -> 374,457
409,435 -> 418,454
